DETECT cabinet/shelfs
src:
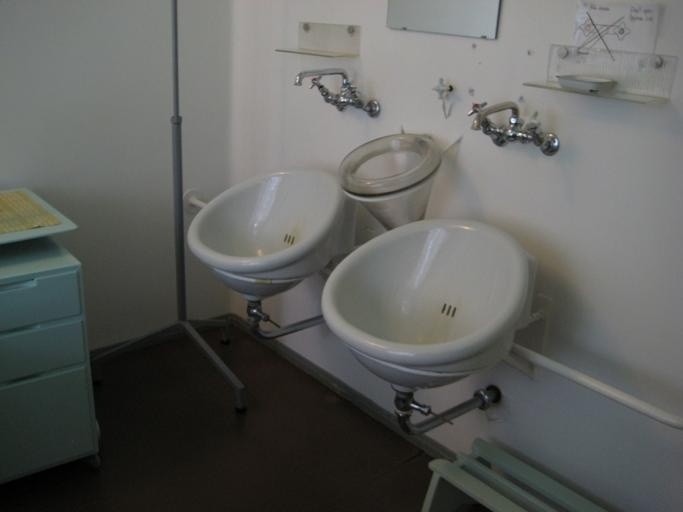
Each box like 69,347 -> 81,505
0,235 -> 105,486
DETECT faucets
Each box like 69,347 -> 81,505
294,69 -> 348,86
469,101 -> 518,132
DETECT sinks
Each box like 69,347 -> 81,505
186,170 -> 351,301
320,219 -> 531,384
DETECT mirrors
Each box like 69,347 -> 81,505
387,0 -> 503,39
